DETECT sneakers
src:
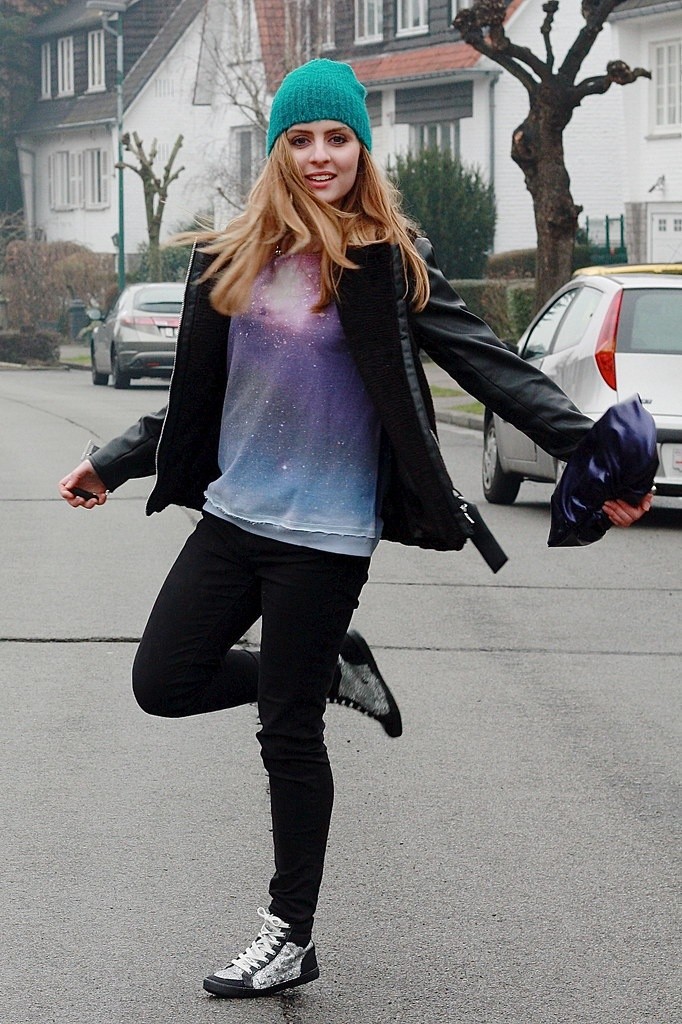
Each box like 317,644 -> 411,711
326,631 -> 402,738
203,906 -> 320,997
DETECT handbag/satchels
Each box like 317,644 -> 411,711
548,394 -> 658,548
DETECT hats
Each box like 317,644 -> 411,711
269,56 -> 374,150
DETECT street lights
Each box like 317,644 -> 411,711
86,0 -> 127,293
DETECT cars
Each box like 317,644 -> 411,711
87,281 -> 187,389
481,262 -> 682,508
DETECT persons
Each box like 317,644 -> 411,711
54,54 -> 658,1002
